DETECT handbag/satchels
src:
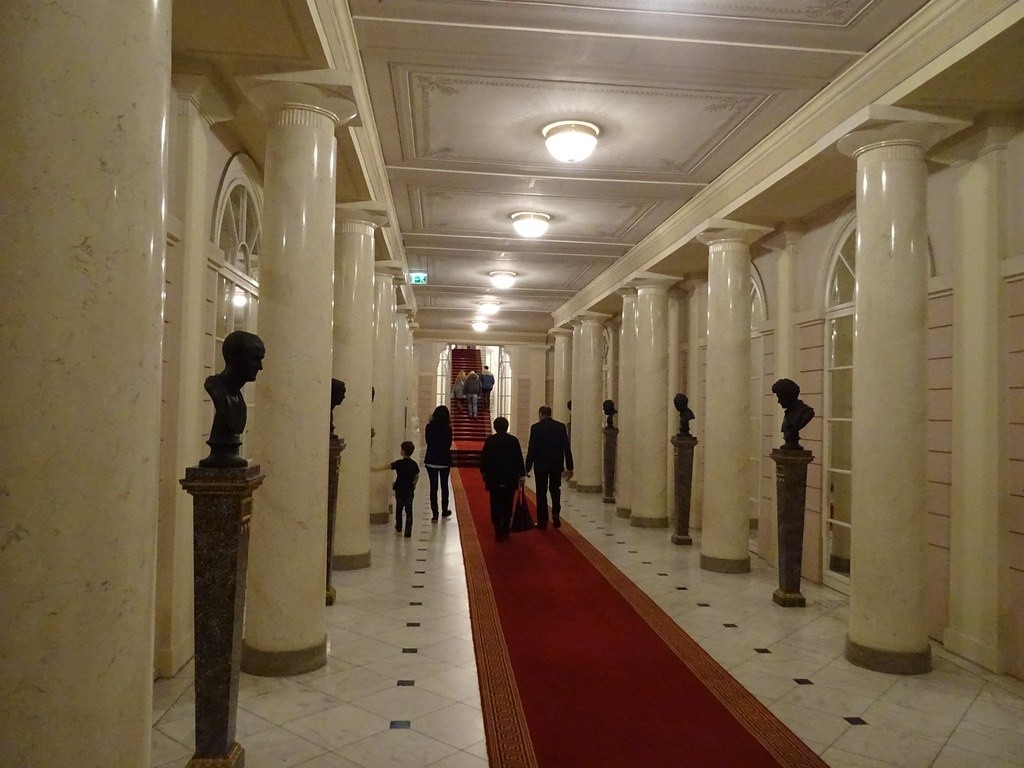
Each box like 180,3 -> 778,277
510,482 -> 534,533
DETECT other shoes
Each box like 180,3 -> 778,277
431,517 -> 438,523
395,526 -> 402,532
533,522 -> 548,530
495,528 -> 510,538
404,530 -> 411,537
552,519 -> 561,529
442,510 -> 451,518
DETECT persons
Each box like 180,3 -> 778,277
603,399 -> 617,416
453,366 -> 495,417
525,406 -> 573,529
567,401 -> 572,410
479,417 -> 526,540
370,441 -> 421,537
674,393 -> 695,421
331,379 -> 346,412
204,330 -> 266,434
771,378 -> 816,432
423,405 -> 453,524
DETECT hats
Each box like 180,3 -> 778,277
457,370 -> 467,379
466,371 -> 479,380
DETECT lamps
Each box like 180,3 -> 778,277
545,123 -> 598,164
513,214 -> 549,239
490,273 -> 516,290
480,303 -> 499,315
472,322 -> 488,332
